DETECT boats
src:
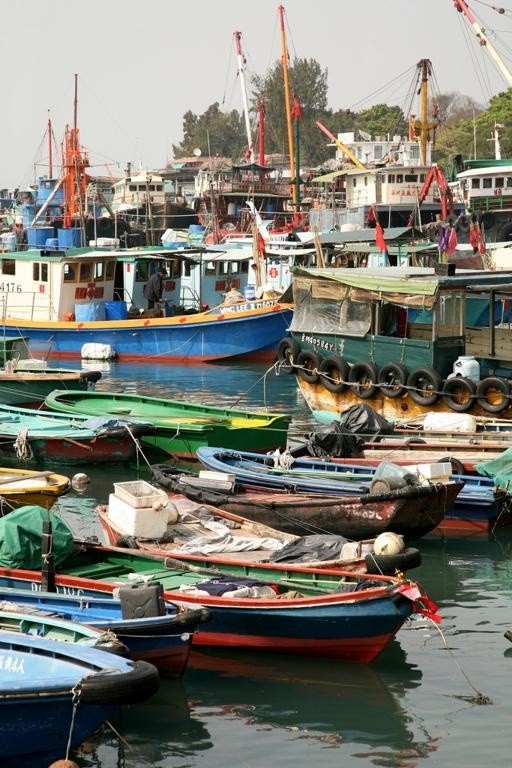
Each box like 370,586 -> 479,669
0,631 -> 158,768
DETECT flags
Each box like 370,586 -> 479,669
375,222 -> 385,253
435,222 -> 484,253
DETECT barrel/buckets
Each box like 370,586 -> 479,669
45,239 -> 58,247
26,226 -> 54,249
421,411 -> 479,435
372,461 -> 410,486
374,532 -> 405,555
75,303 -> 105,321
119,582 -> 165,620
189,224 -> 203,233
58,228 -> 80,247
0,235 -> 16,252
105,300 -> 127,320
453,354 -> 480,383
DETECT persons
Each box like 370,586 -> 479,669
144,267 -> 167,310
